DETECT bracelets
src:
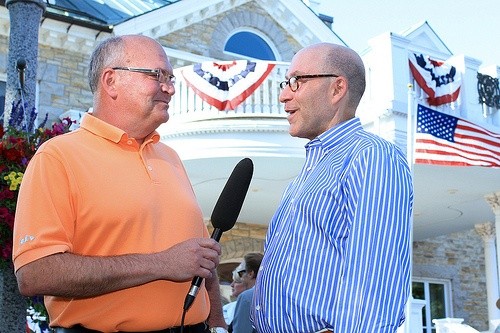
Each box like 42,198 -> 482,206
210,328 -> 229,333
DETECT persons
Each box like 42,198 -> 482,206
252,42 -> 413,333
221,251 -> 264,333
12,34 -> 225,333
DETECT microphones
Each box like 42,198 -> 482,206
183,158 -> 254,312
16,58 -> 27,94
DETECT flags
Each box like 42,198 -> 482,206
415,104 -> 500,167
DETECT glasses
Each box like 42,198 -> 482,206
112,66 -> 176,86
237,269 -> 249,277
279,73 -> 339,91
